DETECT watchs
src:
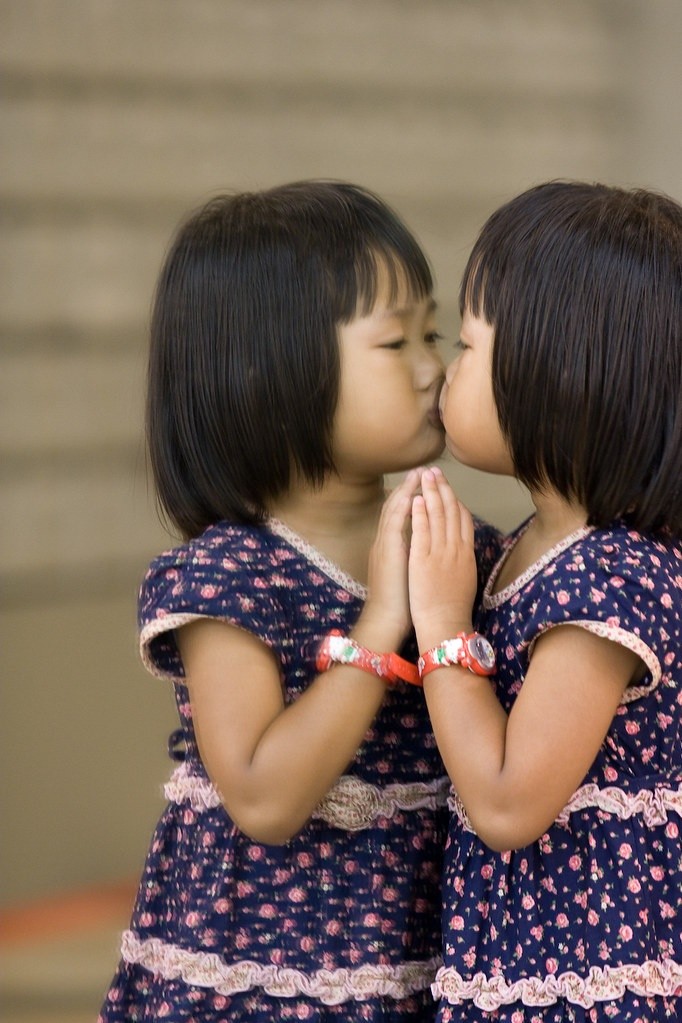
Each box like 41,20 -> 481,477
389,631 -> 497,686
315,628 -> 397,684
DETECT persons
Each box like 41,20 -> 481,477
408,181 -> 682,1023
94,177 -> 506,1022
